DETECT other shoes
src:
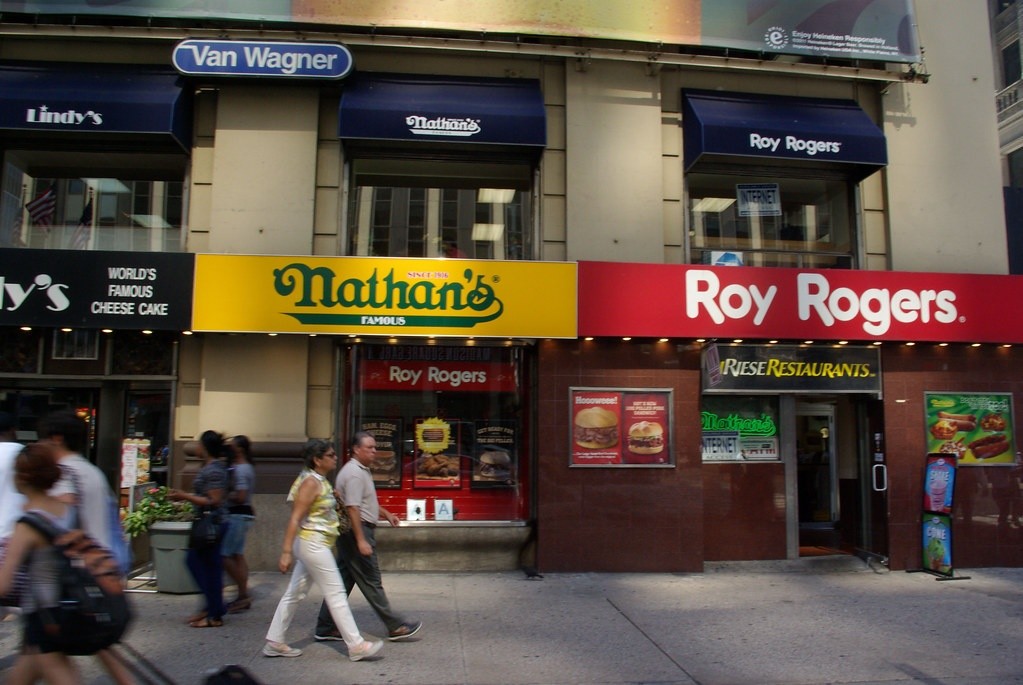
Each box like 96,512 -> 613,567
226,599 -> 249,613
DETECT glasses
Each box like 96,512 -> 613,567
324,451 -> 337,459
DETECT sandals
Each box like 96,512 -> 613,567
189,617 -> 221,627
187,614 -> 207,623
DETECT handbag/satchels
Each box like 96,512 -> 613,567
198,495 -> 223,541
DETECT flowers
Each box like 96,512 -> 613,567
120,485 -> 197,537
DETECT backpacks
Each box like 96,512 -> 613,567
16,513 -> 132,655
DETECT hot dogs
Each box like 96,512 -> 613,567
937,411 -> 977,430
968,433 -> 1009,458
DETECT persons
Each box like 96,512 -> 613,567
437,237 -> 469,260
314,430 -> 422,641
166,430 -> 260,629
0,393 -> 149,685
263,439 -> 385,662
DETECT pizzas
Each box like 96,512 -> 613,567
981,413 -> 1004,431
939,440 -> 966,459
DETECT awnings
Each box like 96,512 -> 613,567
338,75 -> 547,146
0,60 -> 193,157
683,92 -> 888,185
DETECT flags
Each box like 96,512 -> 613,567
11,208 -> 28,249
67,199 -> 93,250
25,187 -> 57,237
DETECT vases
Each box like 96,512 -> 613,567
147,519 -> 226,595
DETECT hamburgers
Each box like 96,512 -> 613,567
479,451 -> 512,477
627,421 -> 664,454
367,451 -> 396,473
573,406 -> 620,448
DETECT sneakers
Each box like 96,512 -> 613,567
348,640 -> 383,662
314,631 -> 343,641
387,622 -> 421,641
262,643 -> 303,657
